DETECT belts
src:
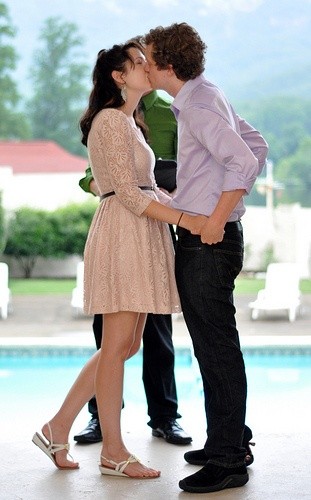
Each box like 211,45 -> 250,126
176,221 -> 243,236
100,186 -> 155,202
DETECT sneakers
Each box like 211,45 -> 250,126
179,458 -> 249,493
184,439 -> 255,467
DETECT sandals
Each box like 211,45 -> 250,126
31,421 -> 80,469
98,454 -> 140,477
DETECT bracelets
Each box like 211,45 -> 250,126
177,212 -> 183,227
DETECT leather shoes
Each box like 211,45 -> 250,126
74,415 -> 103,443
152,420 -> 192,444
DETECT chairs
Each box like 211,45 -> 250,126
0,262 -> 12,318
249,262 -> 303,323
71,261 -> 84,316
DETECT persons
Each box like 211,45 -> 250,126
74,33 -> 195,446
31,43 -> 225,478
144,22 -> 269,494
